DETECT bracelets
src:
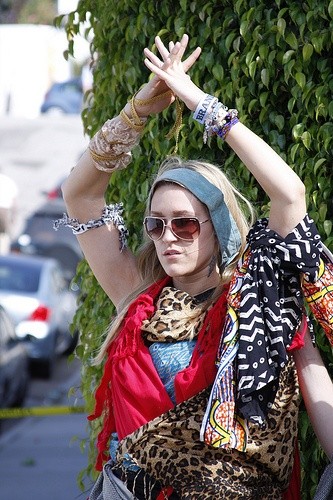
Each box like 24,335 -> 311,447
192,92 -> 240,140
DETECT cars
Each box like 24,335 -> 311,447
0,254 -> 80,380
0,308 -> 27,413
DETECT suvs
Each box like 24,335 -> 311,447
8,181 -> 84,286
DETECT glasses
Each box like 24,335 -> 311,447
143,216 -> 211,241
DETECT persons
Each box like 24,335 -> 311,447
292,242 -> 333,500
52,32 -> 320,500
